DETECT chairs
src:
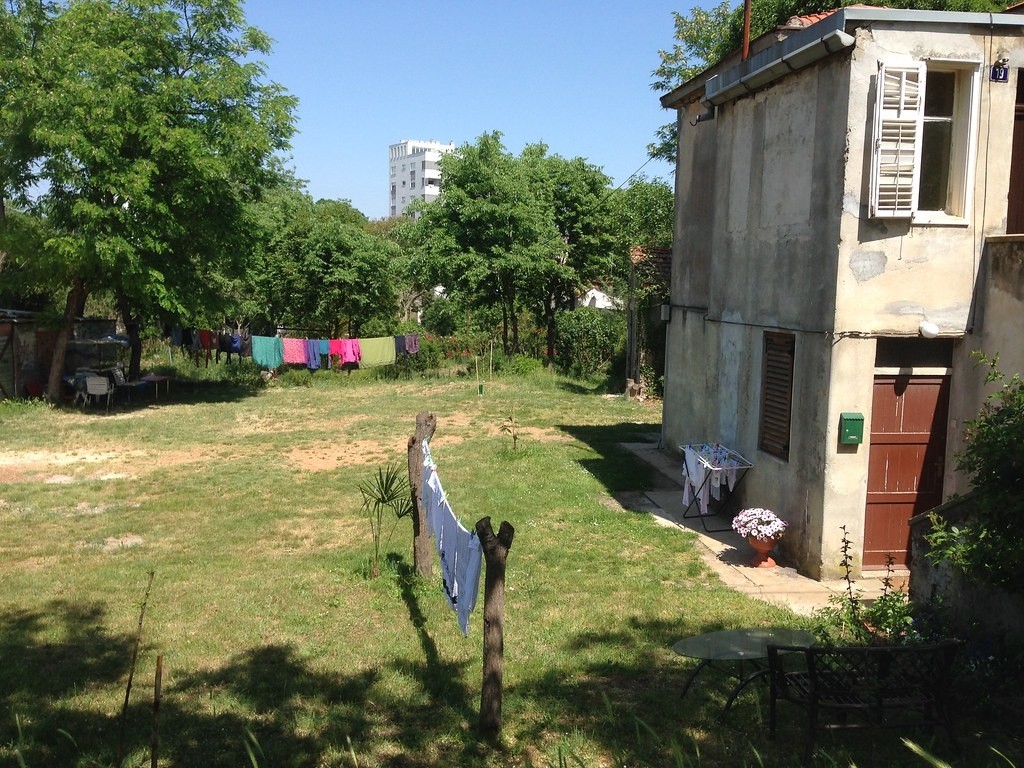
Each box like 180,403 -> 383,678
762,643 -> 967,763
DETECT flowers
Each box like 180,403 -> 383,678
732,507 -> 789,541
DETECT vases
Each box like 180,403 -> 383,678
747,533 -> 779,569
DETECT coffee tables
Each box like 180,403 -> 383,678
673,627 -> 817,725
